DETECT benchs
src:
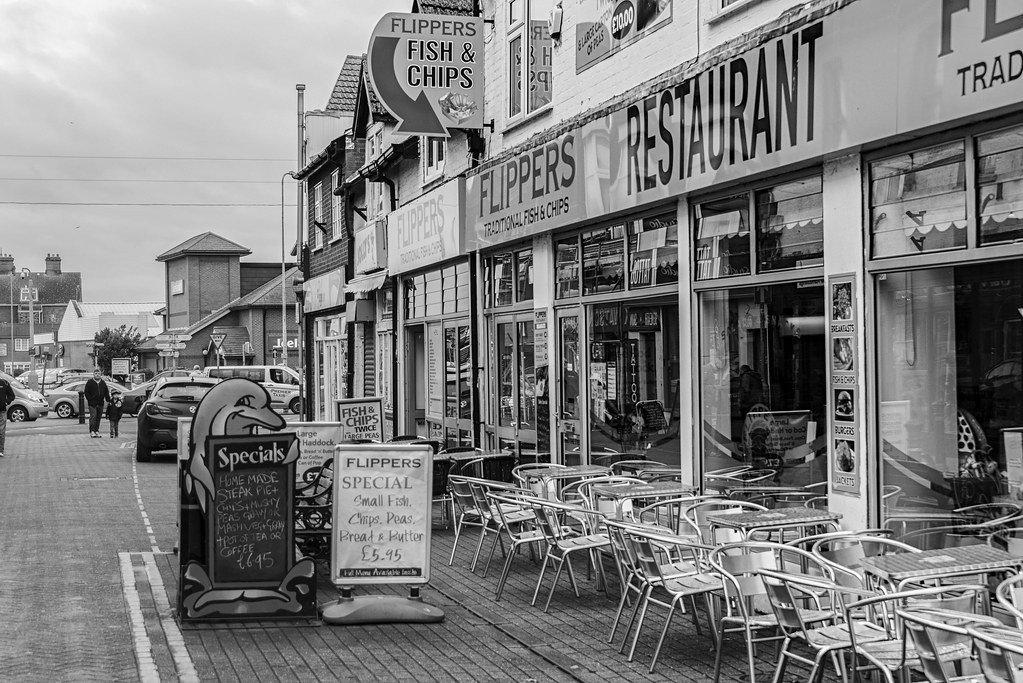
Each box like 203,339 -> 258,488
290,457 -> 335,576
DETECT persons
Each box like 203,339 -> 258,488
0,374 -> 16,456
189,365 -> 204,377
738,364 -> 763,418
84,369 -> 110,437
106,390 -> 122,438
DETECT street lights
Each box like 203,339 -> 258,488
21,267 -> 38,394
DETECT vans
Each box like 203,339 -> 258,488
202,365 -> 307,413
0,369 -> 50,424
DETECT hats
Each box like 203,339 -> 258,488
111,388 -> 120,394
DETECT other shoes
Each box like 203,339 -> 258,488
115,436 -> 118,438
90,431 -> 102,437
111,435 -> 113,438
0,452 -> 4,457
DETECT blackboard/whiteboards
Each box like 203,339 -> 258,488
329,443 -> 432,582
636,401 -> 668,431
206,432 -> 296,589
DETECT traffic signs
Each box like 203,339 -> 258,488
157,350 -> 179,358
155,334 -> 173,341
155,344 -> 169,350
170,343 -> 187,350
174,333 -> 192,341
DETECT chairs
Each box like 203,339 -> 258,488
337,434 -> 1023,683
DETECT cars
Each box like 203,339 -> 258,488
15,367 -> 122,389
119,380 -> 158,418
45,379 -> 129,420
141,368 -> 202,389
134,373 -> 235,465
